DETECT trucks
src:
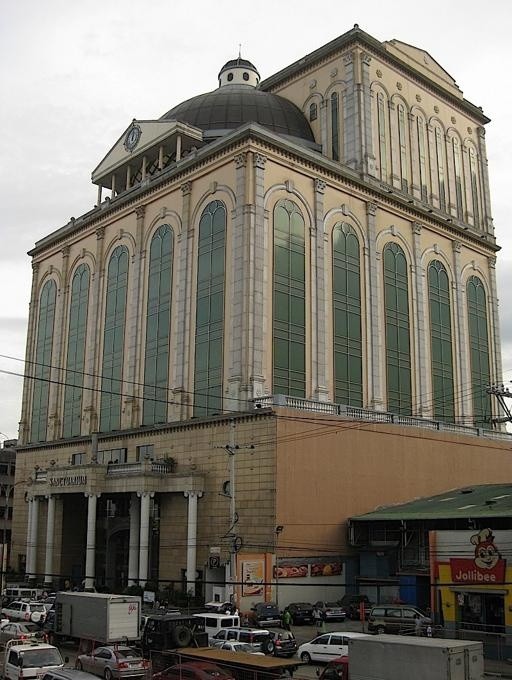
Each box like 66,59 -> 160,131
40,591 -> 141,653
131,613 -> 308,680
316,633 -> 485,680
0,639 -> 69,680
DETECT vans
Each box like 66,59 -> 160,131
192,613 -> 240,643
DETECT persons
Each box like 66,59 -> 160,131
412,613 -> 423,636
312,607 -> 323,627
243,613 -> 249,627
282,609 -> 294,633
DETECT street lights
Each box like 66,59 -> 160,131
274,526 -> 284,606
1,477 -> 48,595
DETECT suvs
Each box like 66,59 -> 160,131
208,627 -> 274,657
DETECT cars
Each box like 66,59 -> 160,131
250,594 -> 372,628
41,666 -> 102,680
297,631 -> 373,665
0,588 -> 56,645
75,645 -> 236,680
368,604 -> 432,633
260,627 -> 299,658
